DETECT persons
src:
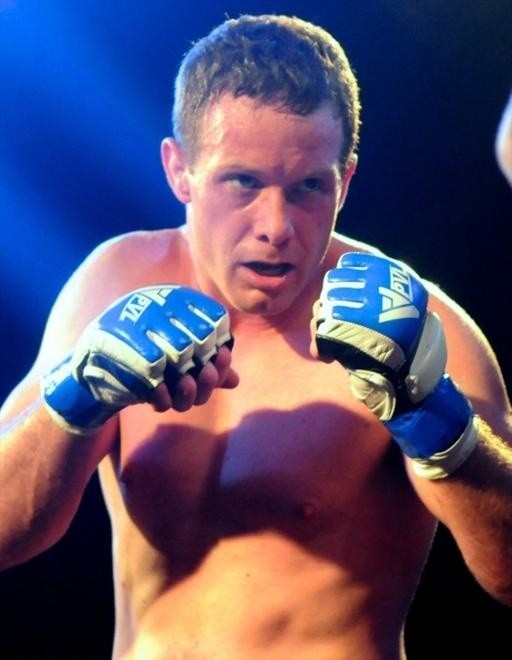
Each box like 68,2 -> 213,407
0,9 -> 511,659
